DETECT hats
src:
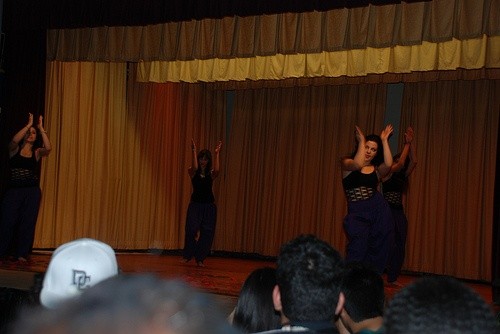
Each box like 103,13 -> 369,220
40,239 -> 118,309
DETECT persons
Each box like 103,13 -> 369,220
341,124 -> 417,288
225,234 -> 500,334
0,113 -> 51,265
0,272 -> 237,334
35,237 -> 119,310
181,138 -> 222,267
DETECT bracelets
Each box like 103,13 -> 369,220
40,131 -> 47,134
26,126 -> 29,129
